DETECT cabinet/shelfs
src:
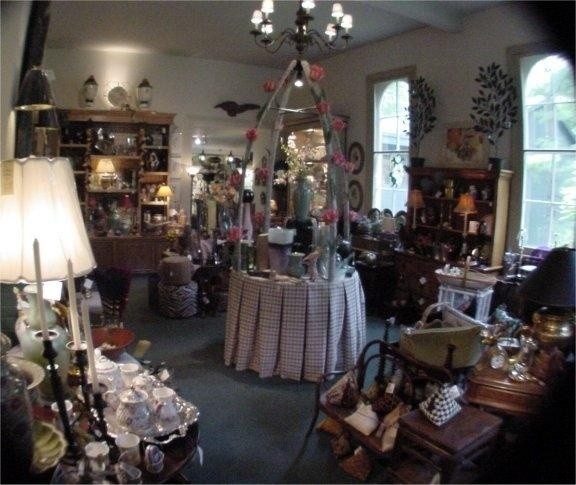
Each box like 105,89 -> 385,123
405,165 -> 514,314
56,108 -> 177,275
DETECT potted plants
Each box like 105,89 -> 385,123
405,76 -> 434,169
472,62 -> 518,171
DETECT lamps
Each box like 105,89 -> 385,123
81,75 -> 99,105
520,247 -> 576,343
135,77 -> 152,107
250,1 -> 354,56
1,157 -> 98,313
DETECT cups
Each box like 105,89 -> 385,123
152,387 -> 179,423
86,441 -> 111,470
51,400 -> 74,430
116,433 -> 142,467
120,363 -> 139,388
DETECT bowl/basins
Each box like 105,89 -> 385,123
88,327 -> 135,360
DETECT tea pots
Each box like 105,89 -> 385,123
101,388 -> 166,432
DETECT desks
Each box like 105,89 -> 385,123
224,259 -> 367,382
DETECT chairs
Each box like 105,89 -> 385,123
277,340 -> 448,484
